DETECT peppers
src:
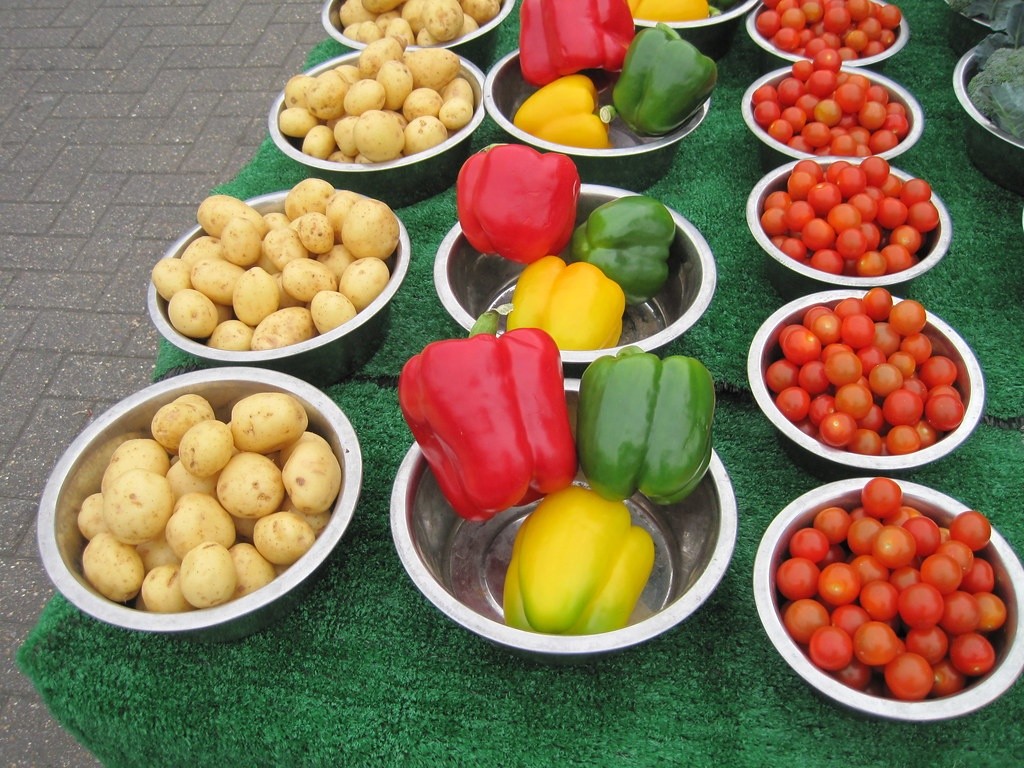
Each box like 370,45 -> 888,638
399,0 -> 715,636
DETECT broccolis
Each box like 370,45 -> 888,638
950,0 -> 1024,143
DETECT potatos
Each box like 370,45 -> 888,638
78,0 -> 498,612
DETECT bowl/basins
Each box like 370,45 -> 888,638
148,191 -> 411,382
753,476 -> 1024,720
389,377 -> 737,654
745,0 -> 910,66
740,66 -> 925,160
482,46 -> 712,158
951,45 -> 1024,195
747,290 -> 985,468
745,155 -> 953,287
266,51 -> 488,190
632,1 -> 760,50
36,364 -> 364,644
432,182 -> 717,362
320,0 -> 515,50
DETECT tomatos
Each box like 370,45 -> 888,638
753,0 -> 1008,700
